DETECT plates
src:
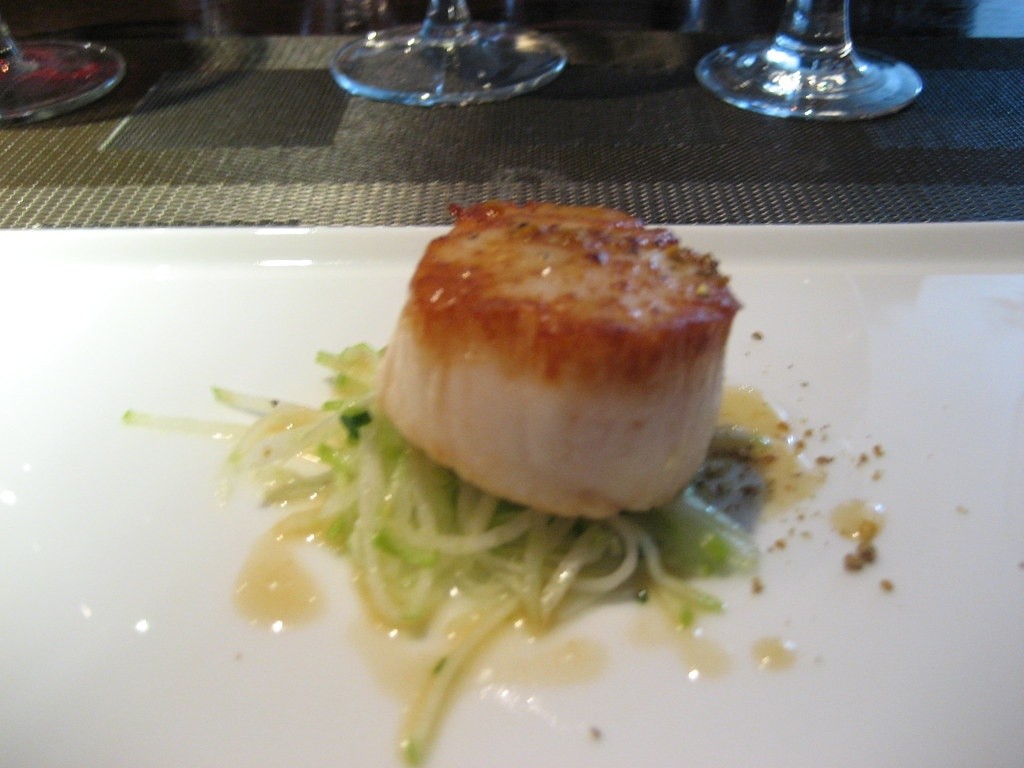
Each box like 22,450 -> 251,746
0,224 -> 1024,768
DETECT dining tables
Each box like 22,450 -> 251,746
0,38 -> 1024,768
